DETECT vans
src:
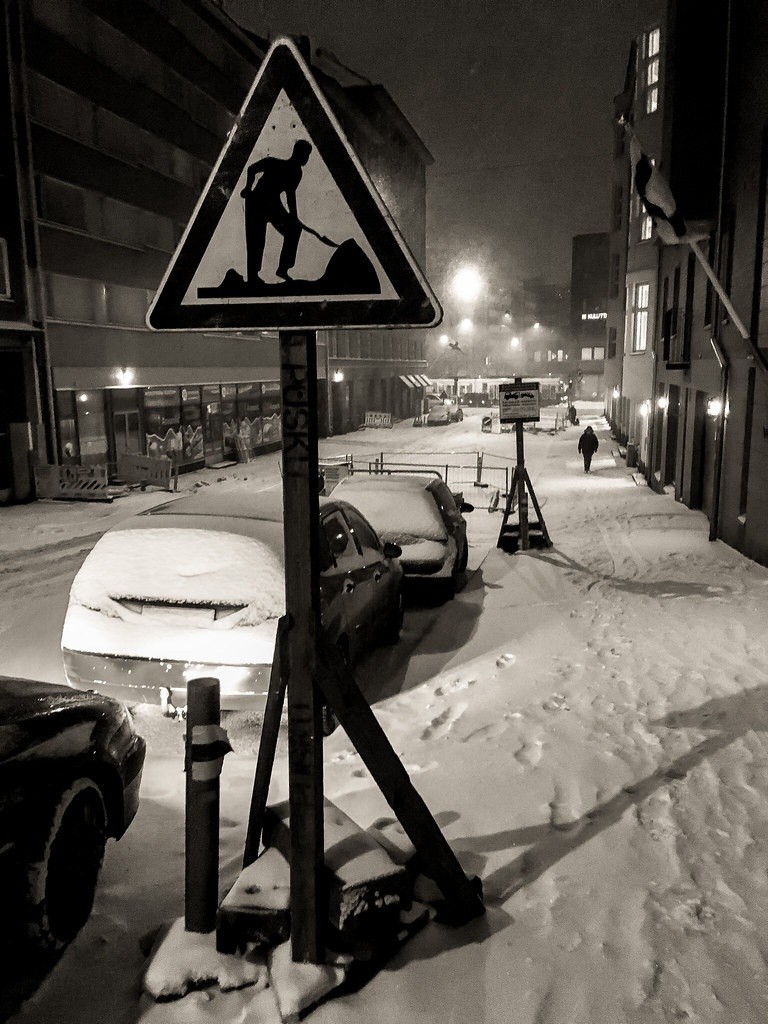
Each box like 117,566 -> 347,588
457,393 -> 490,407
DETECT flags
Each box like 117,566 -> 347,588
629,138 -> 690,244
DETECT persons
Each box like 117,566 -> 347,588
577,426 -> 599,472
568,404 -> 578,424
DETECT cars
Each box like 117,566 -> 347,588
427,404 -> 452,425
55,482 -> 409,735
426,394 -> 454,409
0,676 -> 148,965
448,405 -> 463,423
329,474 -> 475,597
